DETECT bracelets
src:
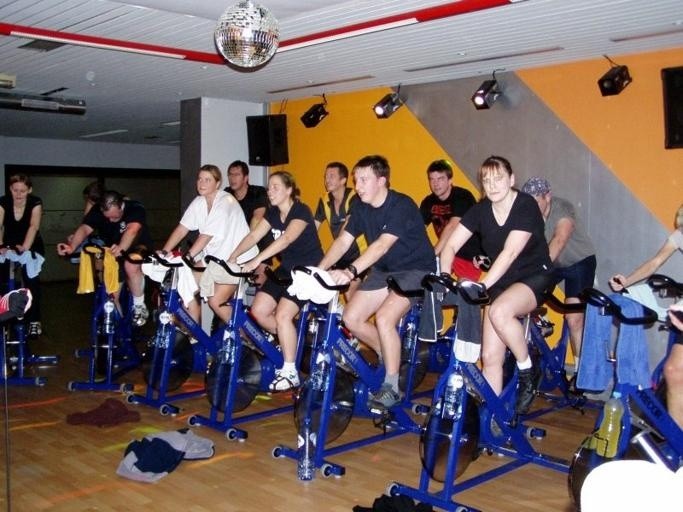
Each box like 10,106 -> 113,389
344,265 -> 358,277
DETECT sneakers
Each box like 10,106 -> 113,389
132,303 -> 149,328
514,364 -> 541,415
370,382 -> 401,411
28,321 -> 43,336
268,367 -> 301,392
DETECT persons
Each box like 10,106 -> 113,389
420,159 -> 476,255
0,174 -> 44,336
223,160 -> 275,289
439,154 -> 557,415
83,181 -> 105,215
521,177 -> 597,372
609,205 -> 683,433
313,162 -> 367,302
225,170 -> 324,390
160,165 -> 259,326
58,192 -> 152,326
315,155 -> 437,410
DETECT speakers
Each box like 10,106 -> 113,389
659,66 -> 683,150
246,114 -> 288,167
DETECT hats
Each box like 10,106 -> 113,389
521,177 -> 551,200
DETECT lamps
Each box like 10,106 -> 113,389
214,0 -> 283,69
299,102 -> 329,129
597,65 -> 632,97
471,79 -> 503,110
373,93 -> 403,119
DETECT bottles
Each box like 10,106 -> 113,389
597,392 -> 625,457
313,339 -> 331,390
104,296 -> 114,333
221,320 -> 236,366
156,310 -> 170,349
402,315 -> 416,351
443,356 -> 464,420
